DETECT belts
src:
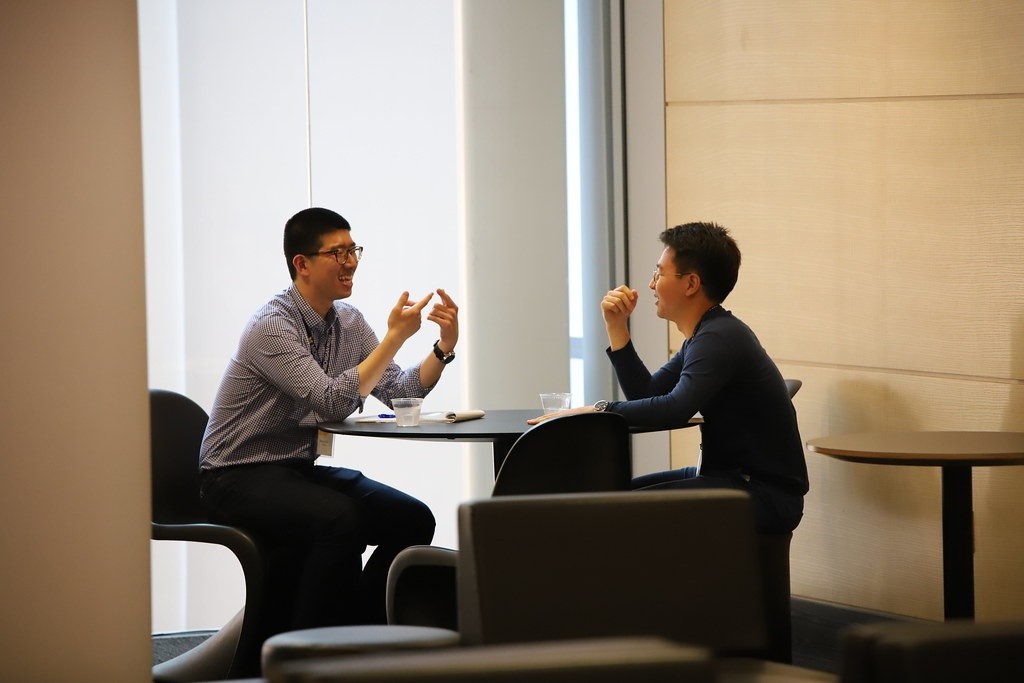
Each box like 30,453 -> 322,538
200,464 -> 314,475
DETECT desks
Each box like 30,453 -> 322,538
315,408 -> 705,525
808,428 -> 1024,618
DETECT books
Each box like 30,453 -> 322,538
357,410 -> 485,423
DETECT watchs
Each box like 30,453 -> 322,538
594,400 -> 609,411
433,340 -> 455,364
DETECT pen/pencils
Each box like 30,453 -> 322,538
378,414 -> 396,418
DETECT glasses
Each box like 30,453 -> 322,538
290,246 -> 363,265
653,267 -> 704,286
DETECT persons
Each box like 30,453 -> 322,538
198,207 -> 459,658
527,222 -> 810,534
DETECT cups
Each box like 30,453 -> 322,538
540,393 -> 572,415
391,398 -> 423,427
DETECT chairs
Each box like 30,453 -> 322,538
150,388 -> 297,680
387,410 -> 631,630
